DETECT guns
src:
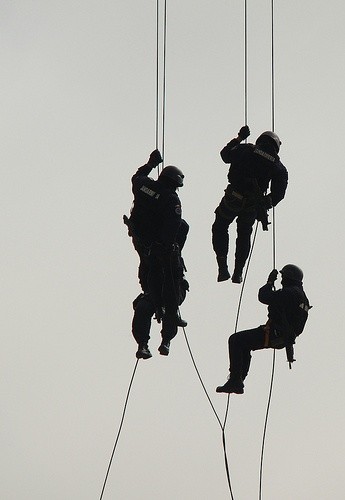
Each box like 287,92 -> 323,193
286,345 -> 296,369
259,212 -> 271,233
122,215 -> 129,227
153,311 -> 163,324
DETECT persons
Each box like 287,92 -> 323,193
216,264 -> 309,395
213,125 -> 288,283
128,150 -> 189,358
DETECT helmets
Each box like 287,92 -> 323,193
255,131 -> 280,154
279,264 -> 304,283
151,238 -> 176,261
161,165 -> 184,187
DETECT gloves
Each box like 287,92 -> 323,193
237,125 -> 250,140
267,269 -> 278,287
148,149 -> 163,168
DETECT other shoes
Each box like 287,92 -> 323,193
216,378 -> 245,394
177,316 -> 187,327
217,268 -> 230,282
159,342 -> 168,354
232,272 -> 243,283
136,347 -> 151,359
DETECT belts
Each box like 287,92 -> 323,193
231,191 -> 243,200
